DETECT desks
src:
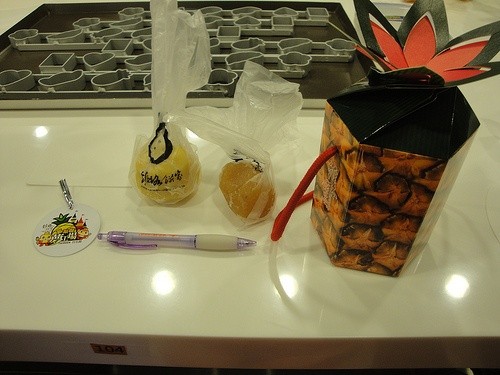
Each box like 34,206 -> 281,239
1,1 -> 500,375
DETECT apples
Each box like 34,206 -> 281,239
133,140 -> 201,205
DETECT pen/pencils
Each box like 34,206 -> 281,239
96,228 -> 260,256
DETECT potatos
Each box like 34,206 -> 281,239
219,160 -> 275,220
